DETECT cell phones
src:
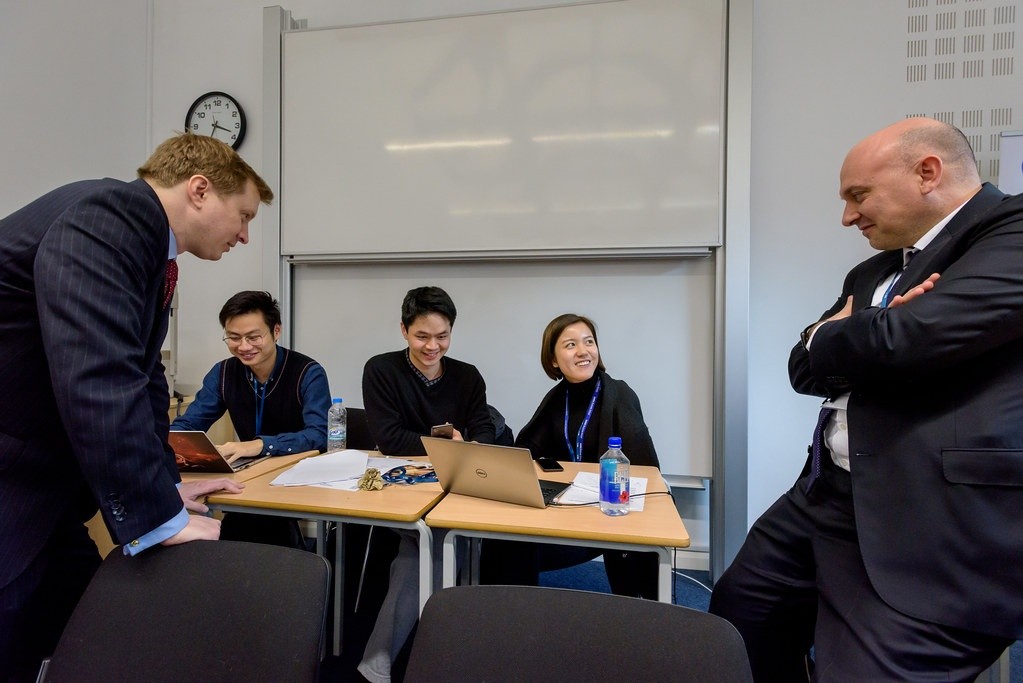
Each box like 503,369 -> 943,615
431,424 -> 453,439
535,458 -> 564,472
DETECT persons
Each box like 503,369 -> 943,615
168,290 -> 333,551
356,287 -> 496,683
479,313 -> 661,600
0,134 -> 275,683
711,117 -> 1023,683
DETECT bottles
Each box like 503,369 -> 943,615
598,436 -> 630,516
327,398 -> 347,453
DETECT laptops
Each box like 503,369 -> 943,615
168,431 -> 272,473
420,435 -> 574,508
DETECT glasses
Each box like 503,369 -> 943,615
222,328 -> 270,347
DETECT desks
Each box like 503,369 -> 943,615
82,450 -> 320,558
424,457 -> 689,600
210,455 -> 456,660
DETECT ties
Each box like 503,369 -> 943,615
161,258 -> 180,318
805,409 -> 831,496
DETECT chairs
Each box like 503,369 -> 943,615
345,408 -> 377,450
42,543 -> 332,682
401,583 -> 755,682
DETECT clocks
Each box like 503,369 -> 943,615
183,91 -> 245,151
